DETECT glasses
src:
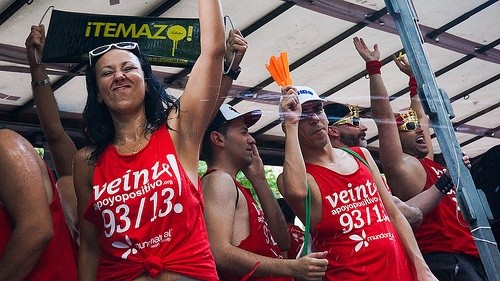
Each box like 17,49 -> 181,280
333,115 -> 361,127
398,120 -> 421,130
89,41 -> 141,66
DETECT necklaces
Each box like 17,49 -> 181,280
115,130 -> 147,153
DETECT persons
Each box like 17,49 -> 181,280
0,129 -> 80,281
276,85 -> 440,281
199,103 -> 329,281
323,103 -> 472,232
25,24 -> 80,247
73,0 -> 227,281
276,198 -> 305,259
353,36 -> 489,281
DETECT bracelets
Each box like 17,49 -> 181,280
366,60 -> 383,76
434,173 -> 454,196
31,77 -> 50,86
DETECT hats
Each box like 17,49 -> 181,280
204,103 -> 261,128
323,100 -> 361,127
279,85 -> 328,122
393,107 -> 420,127
